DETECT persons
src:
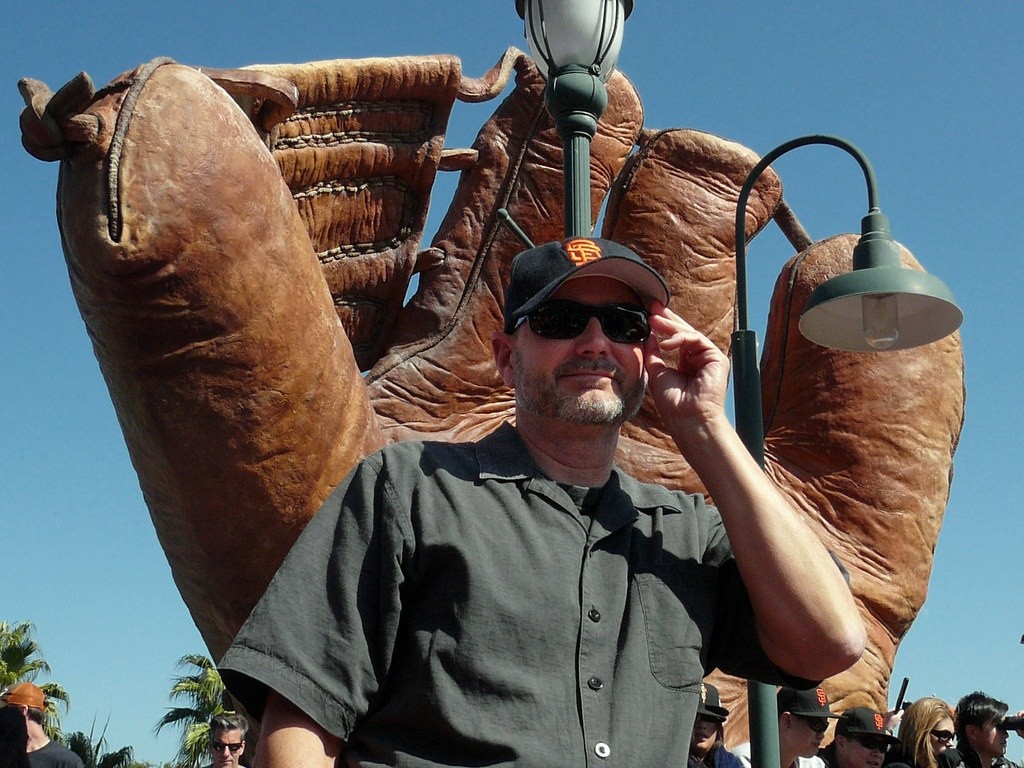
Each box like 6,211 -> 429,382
0,682 -> 84,767
218,234 -> 866,768
202,713 -> 248,768
686,683 -> 1023,768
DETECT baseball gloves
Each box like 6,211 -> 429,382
13,37 -> 973,767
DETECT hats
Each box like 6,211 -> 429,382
834,707 -> 903,746
505,237 -> 670,338
0,682 -> 45,711
777,684 -> 848,719
697,682 -> 729,723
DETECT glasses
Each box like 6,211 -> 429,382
842,733 -> 888,754
511,298 -> 653,343
696,713 -> 723,725
211,735 -> 244,751
930,728 -> 960,749
791,714 -> 830,734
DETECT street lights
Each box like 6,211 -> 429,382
727,132 -> 968,768
493,0 -> 637,248
